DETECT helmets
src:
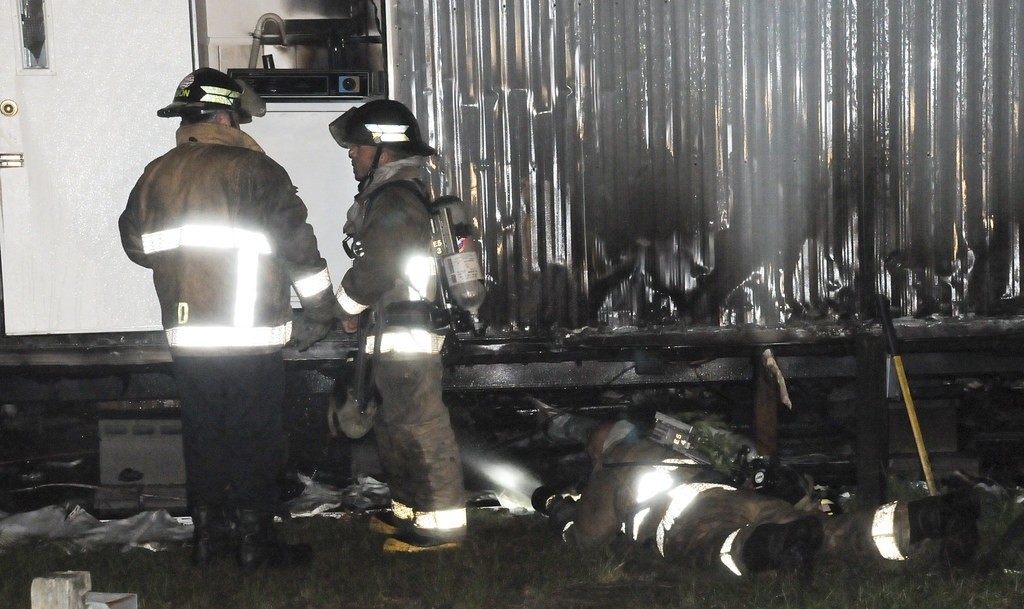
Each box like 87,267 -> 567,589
156,67 -> 266,124
328,101 -> 436,157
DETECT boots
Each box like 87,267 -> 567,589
186,500 -> 239,562
234,506 -> 311,567
742,517 -> 824,601
909,488 -> 980,569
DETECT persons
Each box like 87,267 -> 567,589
118,69 -> 333,567
528,417 -> 982,588
332,99 -> 471,550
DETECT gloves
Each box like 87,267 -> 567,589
549,495 -> 578,531
292,314 -> 333,352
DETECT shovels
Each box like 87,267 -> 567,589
338,309 -> 376,440
870,294 -> 980,546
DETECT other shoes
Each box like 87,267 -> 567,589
369,511 -> 415,535
382,530 -> 457,553
